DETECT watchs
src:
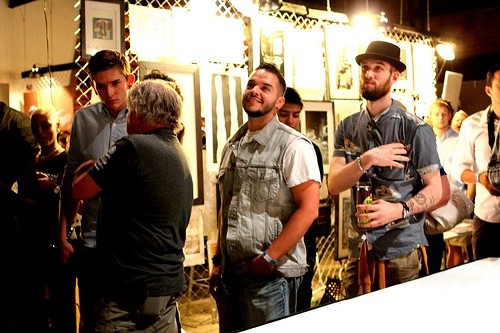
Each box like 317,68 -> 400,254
52,185 -> 61,194
399,200 -> 410,219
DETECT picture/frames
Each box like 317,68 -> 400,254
252,16 -> 293,89
334,188 -> 351,260
81,0 -> 125,59
301,102 -> 333,175
138,62 -> 204,209
181,216 -> 205,267
389,40 -> 414,90
325,26 -> 361,101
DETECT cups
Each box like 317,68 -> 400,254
356,186 -> 373,224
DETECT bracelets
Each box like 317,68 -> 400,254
262,252 -> 278,265
356,155 -> 368,174
475,173 -> 480,184
212,254 -> 222,263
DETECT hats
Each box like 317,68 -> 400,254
355,41 -> 406,74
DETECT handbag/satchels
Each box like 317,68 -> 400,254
305,198 -> 332,236
425,182 -> 474,234
487,160 -> 500,190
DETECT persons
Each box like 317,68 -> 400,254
53,50 -> 141,333
73,80 -> 192,333
142,69 -> 184,149
0,100 -> 77,333
208,63 -> 322,333
412,62 -> 500,270
272,84 -> 325,312
325,40 -> 451,299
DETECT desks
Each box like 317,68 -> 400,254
235,257 -> 500,333
